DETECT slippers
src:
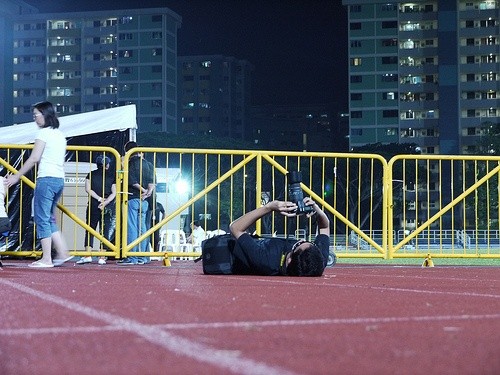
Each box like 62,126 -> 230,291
28,262 -> 54,268
53,256 -> 74,266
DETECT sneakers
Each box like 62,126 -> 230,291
76,256 -> 92,263
98,259 -> 106,265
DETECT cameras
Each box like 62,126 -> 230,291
286,171 -> 314,215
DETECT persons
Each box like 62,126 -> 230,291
186,219 -> 207,248
117,140 -> 166,265
76,155 -> 116,264
0,101 -> 75,267
229,197 -> 330,277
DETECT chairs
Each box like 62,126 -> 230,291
159,229 -> 226,261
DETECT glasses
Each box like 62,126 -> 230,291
293,241 -> 316,252
33,112 -> 43,120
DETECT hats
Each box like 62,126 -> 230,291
96,155 -> 111,165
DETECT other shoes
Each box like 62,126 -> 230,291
138,261 -> 144,265
117,260 -> 132,264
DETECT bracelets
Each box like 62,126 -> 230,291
98,197 -> 101,202
